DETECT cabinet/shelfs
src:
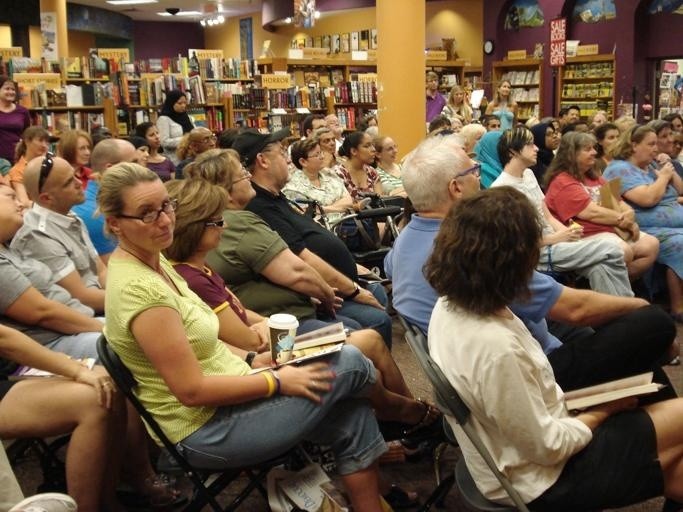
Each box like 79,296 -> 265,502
492,57 -> 544,126
555,53 -> 616,124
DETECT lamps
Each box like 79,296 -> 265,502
199,4 -> 225,27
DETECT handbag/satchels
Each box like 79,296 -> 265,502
599,176 -> 635,241
267,462 -> 394,512
335,205 -> 381,254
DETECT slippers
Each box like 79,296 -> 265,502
115,472 -> 189,511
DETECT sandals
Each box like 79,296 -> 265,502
400,400 -> 446,450
382,485 -> 419,511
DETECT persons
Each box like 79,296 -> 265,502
1,78 -> 406,512
384,72 -> 682,512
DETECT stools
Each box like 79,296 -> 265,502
4,434 -> 78,512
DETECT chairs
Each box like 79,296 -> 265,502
96,335 -> 292,511
397,314 -> 458,488
378,196 -> 412,248
404,332 -> 532,512
295,192 -> 400,316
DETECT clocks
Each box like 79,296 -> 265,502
483,40 -> 494,55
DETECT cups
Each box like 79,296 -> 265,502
266,314 -> 299,366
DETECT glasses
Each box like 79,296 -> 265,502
117,200 -> 178,223
191,134 -> 218,144
232,167 -> 252,184
260,145 -> 288,157
205,216 -> 224,227
453,161 -> 481,179
308,150 -> 323,158
38,151 -> 56,194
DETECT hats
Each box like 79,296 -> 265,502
231,125 -> 291,168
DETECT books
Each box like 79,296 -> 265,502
1,58 -> 104,153
111,58 -> 249,135
232,72 -> 377,135
